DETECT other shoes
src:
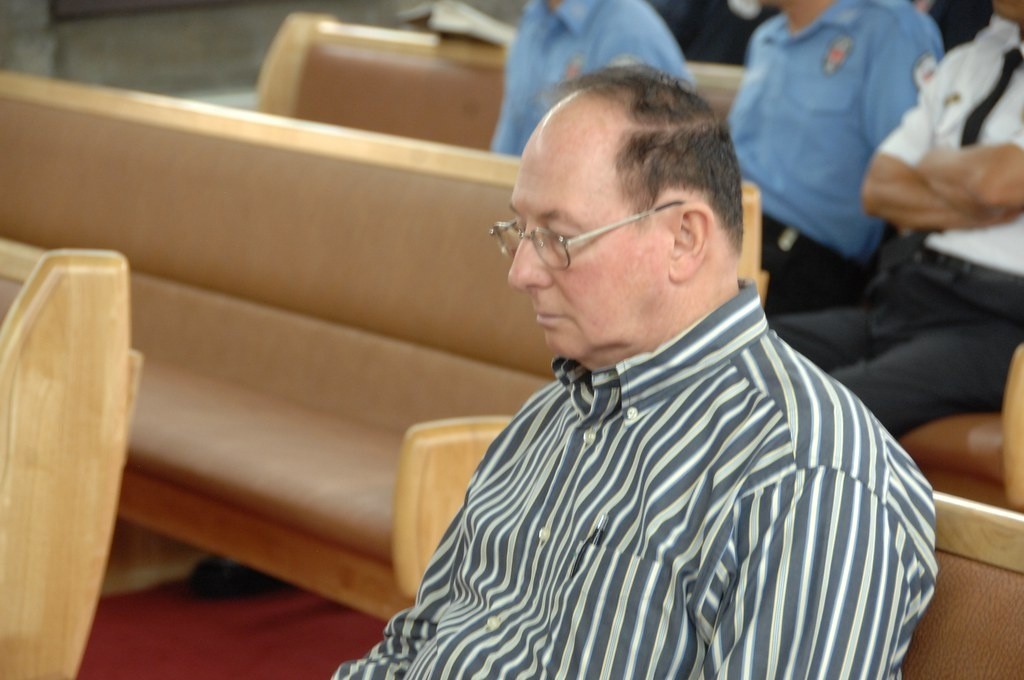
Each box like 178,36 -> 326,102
186,553 -> 297,598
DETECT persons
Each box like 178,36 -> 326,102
492,2 -> 691,158
328,63 -> 938,680
647,1 -> 1023,443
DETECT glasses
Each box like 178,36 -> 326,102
488,201 -> 686,272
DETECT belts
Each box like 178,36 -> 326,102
910,247 -> 1020,286
760,212 -> 844,267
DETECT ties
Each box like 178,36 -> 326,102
881,47 -> 1024,267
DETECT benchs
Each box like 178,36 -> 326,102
1,238 -> 147,680
900,490 -> 1024,678
251,12 -> 1023,511
1,69 -> 770,621
